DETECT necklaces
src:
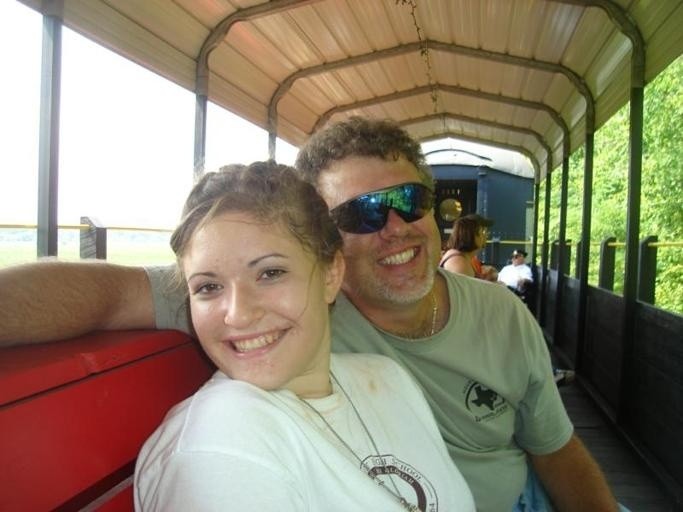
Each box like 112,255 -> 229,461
298,370 -> 422,512
431,292 -> 438,337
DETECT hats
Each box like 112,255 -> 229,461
511,247 -> 529,256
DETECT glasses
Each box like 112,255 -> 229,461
329,181 -> 434,233
512,255 -> 519,259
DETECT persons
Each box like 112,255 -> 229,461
439,214 -> 489,279
496,249 -> 534,303
0,118 -> 633,512
132,158 -> 476,512
